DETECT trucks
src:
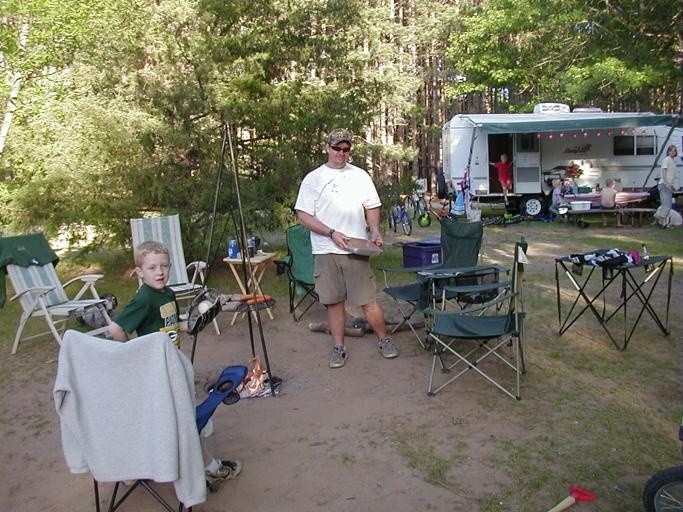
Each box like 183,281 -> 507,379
436,103 -> 683,219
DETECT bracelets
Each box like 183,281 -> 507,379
329,229 -> 334,241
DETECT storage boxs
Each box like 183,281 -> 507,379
401,241 -> 442,268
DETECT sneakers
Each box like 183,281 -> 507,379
378,338 -> 398,358
330,346 -> 347,368
205,460 -> 240,486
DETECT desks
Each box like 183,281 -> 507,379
223,251 -> 277,327
418,264 -> 510,349
554,249 -> 674,353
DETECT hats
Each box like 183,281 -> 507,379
328,128 -> 352,146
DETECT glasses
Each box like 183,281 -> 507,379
329,145 -> 351,153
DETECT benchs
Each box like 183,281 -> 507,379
563,208 -> 658,230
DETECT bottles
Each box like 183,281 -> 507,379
641,244 -> 655,272
246,236 -> 260,258
228,240 -> 237,259
596,183 -> 603,195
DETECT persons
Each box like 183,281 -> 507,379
656,145 -> 677,229
293,128 -> 400,369
109,241 -> 241,482
489,154 -> 513,206
548,177 -> 625,227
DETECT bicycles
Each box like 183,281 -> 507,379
404,185 -> 427,219
418,196 -> 457,228
387,194 -> 413,237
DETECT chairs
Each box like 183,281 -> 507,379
424,242 -> 527,401
2,234 -> 112,355
130,213 -> 221,336
56,330 -> 248,512
274,223 -> 328,322
376,218 -> 484,349
